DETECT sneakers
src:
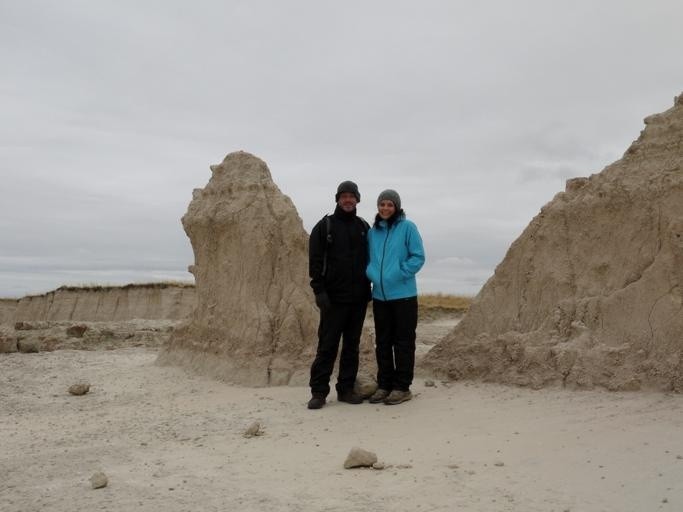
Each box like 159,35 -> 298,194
369,388 -> 413,405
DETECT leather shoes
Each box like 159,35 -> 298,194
337,391 -> 363,404
308,392 -> 326,409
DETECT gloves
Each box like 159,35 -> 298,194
316,291 -> 332,310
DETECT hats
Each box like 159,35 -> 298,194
335,181 -> 360,202
377,189 -> 401,210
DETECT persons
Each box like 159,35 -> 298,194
364,188 -> 425,405
307,179 -> 372,410
